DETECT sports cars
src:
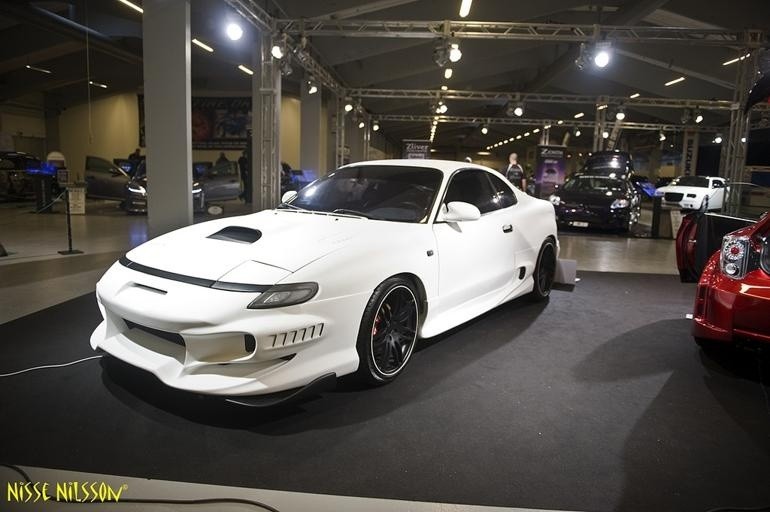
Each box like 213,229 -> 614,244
90,157 -> 559,408
676,210 -> 770,352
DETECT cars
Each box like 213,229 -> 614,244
85,156 -> 243,215
549,150 -> 641,231
653,175 -> 731,214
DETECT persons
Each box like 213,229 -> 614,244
215,152 -> 231,175
236,151 -> 249,204
128,149 -> 141,176
502,153 -> 527,193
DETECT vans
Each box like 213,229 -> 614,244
0,152 -> 60,200
